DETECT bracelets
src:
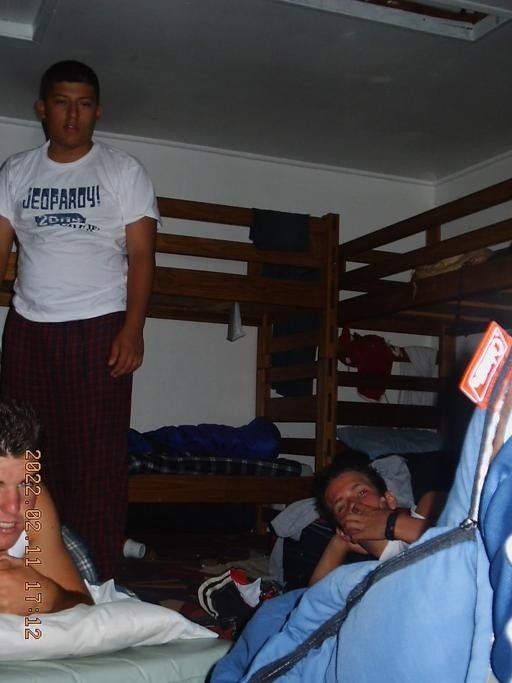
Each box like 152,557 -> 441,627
383,509 -> 404,539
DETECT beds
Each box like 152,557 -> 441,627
0,180 -> 510,682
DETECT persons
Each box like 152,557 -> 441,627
2,58 -> 162,591
309,455 -> 447,582
0,393 -> 97,616
